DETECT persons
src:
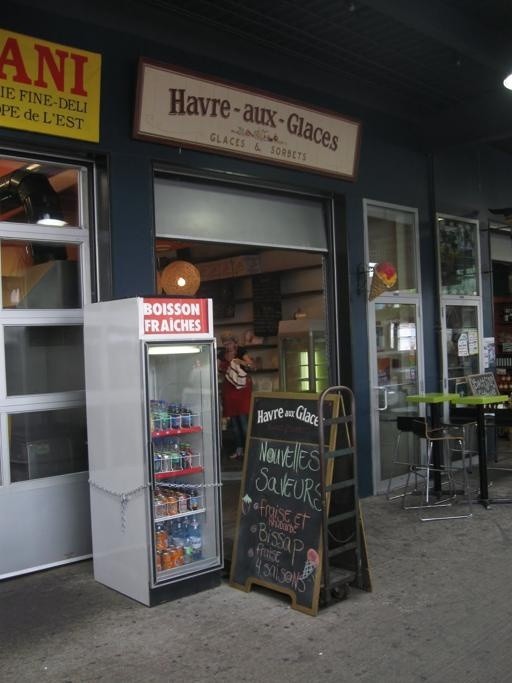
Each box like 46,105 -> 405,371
217,331 -> 261,460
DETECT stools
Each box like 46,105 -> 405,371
385,408 -> 512,523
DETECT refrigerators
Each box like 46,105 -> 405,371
83,295 -> 225,608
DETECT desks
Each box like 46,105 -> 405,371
406,392 -> 511,510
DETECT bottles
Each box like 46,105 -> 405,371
179,442 -> 193,469
150,398 -> 193,431
155,514 -> 203,562
156,481 -> 199,510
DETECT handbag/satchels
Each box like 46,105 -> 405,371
225,360 -> 247,390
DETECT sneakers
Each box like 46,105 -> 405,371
229,452 -> 244,460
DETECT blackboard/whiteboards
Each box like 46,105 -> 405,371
468,372 -> 501,396
229,393 -> 341,617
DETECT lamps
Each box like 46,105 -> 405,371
160,260 -> 202,297
